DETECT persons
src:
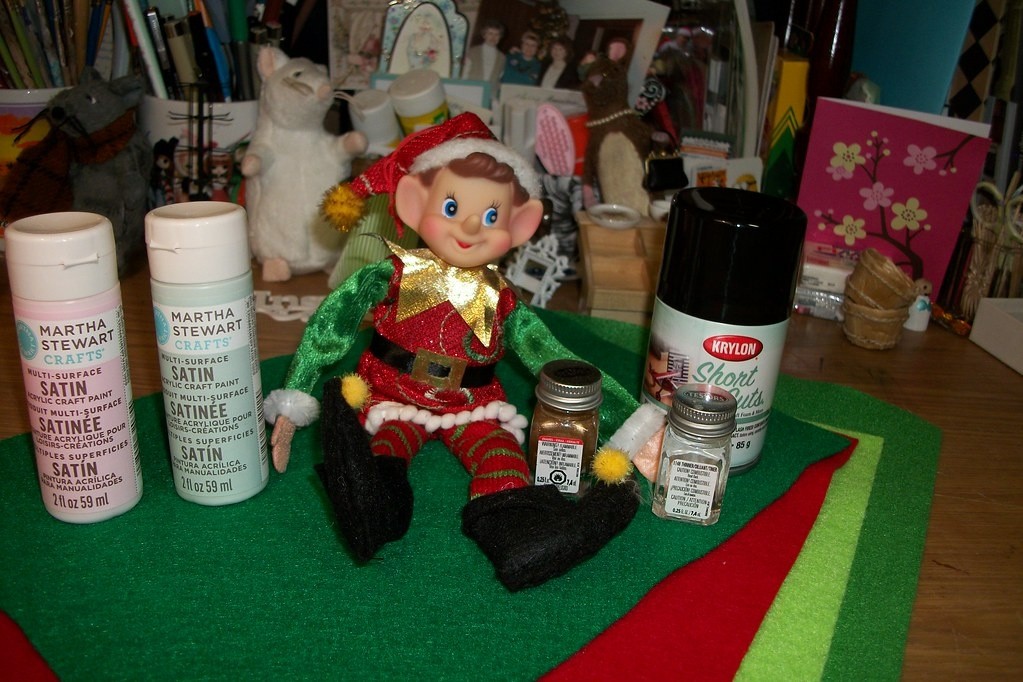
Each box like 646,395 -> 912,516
262,113 -> 666,594
462,20 -> 577,90
659,27 -> 691,53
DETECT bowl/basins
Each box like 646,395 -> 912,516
648,199 -> 672,221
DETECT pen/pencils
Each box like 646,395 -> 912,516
0,0 -> 254,104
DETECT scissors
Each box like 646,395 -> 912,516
970,181 -> 1023,272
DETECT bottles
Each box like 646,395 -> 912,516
5,211 -> 145,525
527,359 -> 604,500
641,184 -> 809,476
348,70 -> 453,156
651,384 -> 738,527
144,201 -> 271,507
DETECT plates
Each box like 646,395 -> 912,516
586,203 -> 644,228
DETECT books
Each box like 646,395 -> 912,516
797,94 -> 992,310
702,0 -> 809,197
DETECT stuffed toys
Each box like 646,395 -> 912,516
578,37 -> 667,216
0,65 -> 156,268
240,47 -> 369,282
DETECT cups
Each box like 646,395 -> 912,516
0,87 -> 75,187
140,96 -> 260,203
